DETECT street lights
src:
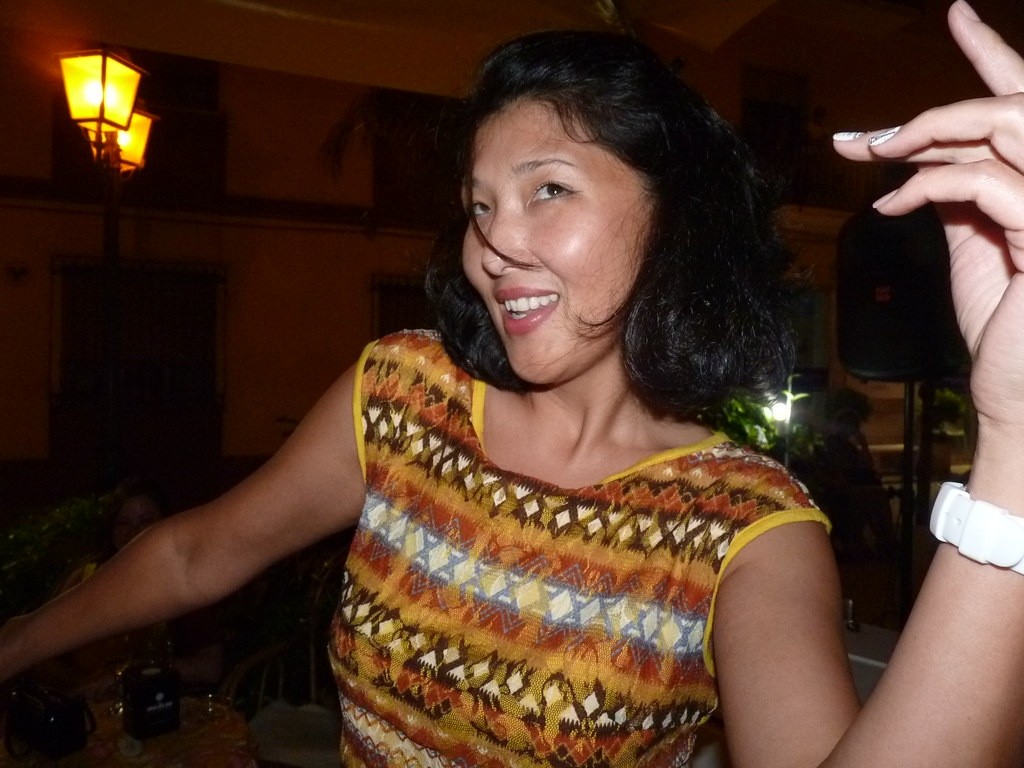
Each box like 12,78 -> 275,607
54,39 -> 163,564
770,365 -> 811,466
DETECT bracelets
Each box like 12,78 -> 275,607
930,481 -> 1024,577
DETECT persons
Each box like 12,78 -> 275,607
795,388 -> 956,629
0,0 -> 1024,768
41,494 -> 227,699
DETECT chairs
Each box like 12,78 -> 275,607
215,548 -> 340,768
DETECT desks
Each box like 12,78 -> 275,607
0,696 -> 251,768
